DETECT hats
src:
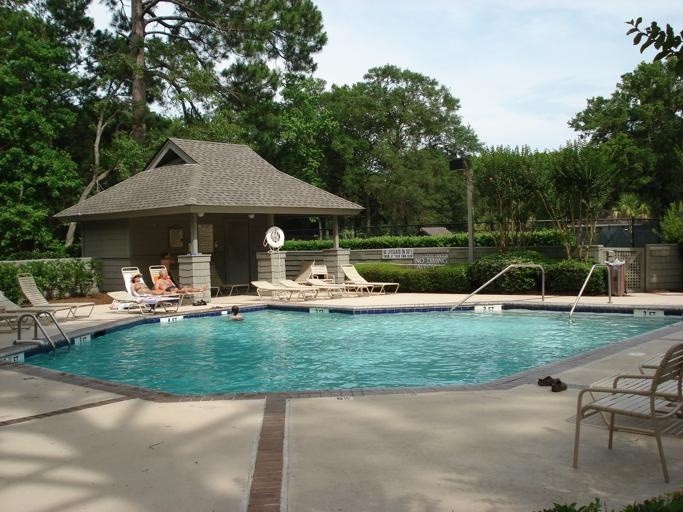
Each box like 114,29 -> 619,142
131,273 -> 142,281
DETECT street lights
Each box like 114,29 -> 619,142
450,158 -> 474,265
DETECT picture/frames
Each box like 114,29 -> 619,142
168,226 -> 185,249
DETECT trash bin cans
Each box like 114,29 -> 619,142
606,260 -> 628,296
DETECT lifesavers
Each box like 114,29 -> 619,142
267,226 -> 284,248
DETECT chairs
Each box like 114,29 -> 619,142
149,264 -> 209,305
210,269 -> 250,296
341,264 -> 399,294
250,281 -> 316,302
122,267 -> 179,311
17,273 -> 95,320
280,279 -> 343,298
169,267 -> 222,298
1,293 -> 70,330
306,279 -> 374,297
107,290 -> 181,315
310,264 -> 336,284
573,338 -> 683,484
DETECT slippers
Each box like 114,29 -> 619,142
538,376 -> 566,392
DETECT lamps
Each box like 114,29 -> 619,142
248,213 -> 255,219
197,213 -> 204,217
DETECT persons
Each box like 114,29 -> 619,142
131,273 -> 179,297
154,269 -> 208,294
229,305 -> 243,320
